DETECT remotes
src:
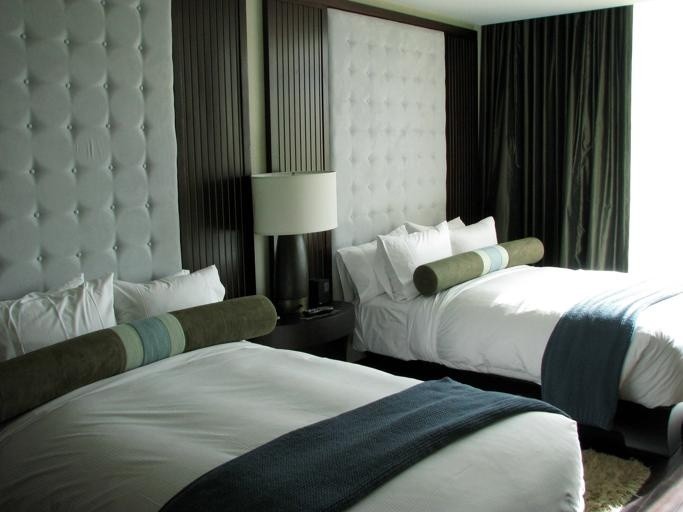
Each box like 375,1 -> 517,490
302,306 -> 334,317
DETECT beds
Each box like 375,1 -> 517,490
0,314 -> 585,512
348,264 -> 683,457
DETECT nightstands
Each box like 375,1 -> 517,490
263,300 -> 355,361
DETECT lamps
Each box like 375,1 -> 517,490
251,170 -> 338,316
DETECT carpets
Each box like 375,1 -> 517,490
584,449 -> 651,512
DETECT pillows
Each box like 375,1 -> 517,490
2,294 -> 278,426
107,264 -> 228,320
335,215 -> 545,306
1,269 -> 120,356
